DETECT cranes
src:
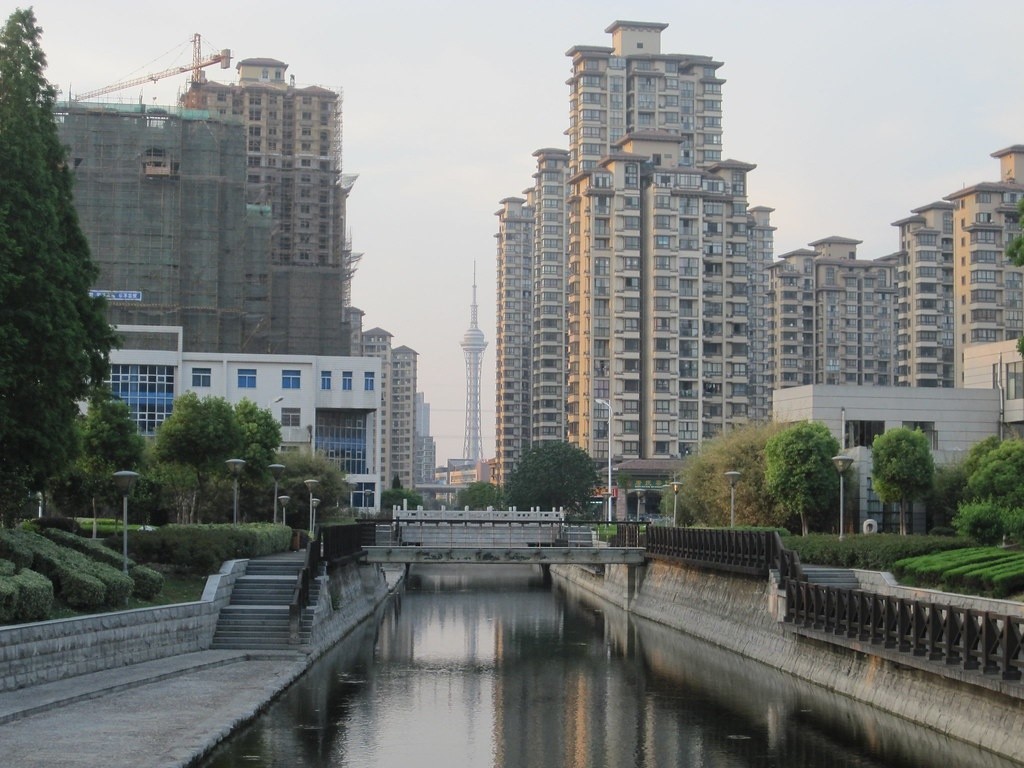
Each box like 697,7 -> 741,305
67,31 -> 236,109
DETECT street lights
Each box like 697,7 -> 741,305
112,471 -> 139,606
278,495 -> 291,526
723,471 -> 741,527
593,398 -> 613,524
311,498 -> 321,533
225,458 -> 246,526
831,456 -> 854,538
601,492 -> 612,529
269,462 -> 285,524
303,478 -> 319,533
363,490 -> 372,512
669,481 -> 684,528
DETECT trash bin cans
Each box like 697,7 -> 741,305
292,529 -> 300,551
